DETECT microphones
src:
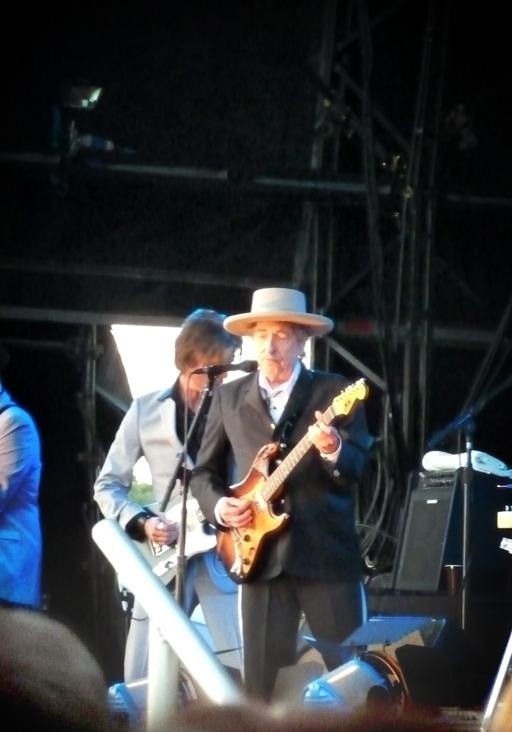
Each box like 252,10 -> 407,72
194,360 -> 259,374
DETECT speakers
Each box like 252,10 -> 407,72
394,484 -> 505,596
341,616 -> 482,706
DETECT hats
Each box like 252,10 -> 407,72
222,286 -> 334,336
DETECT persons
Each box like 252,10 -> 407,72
0,381 -> 46,614
191,285 -> 375,706
92,308 -> 245,690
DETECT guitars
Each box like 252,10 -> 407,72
113,493 -> 218,599
215,378 -> 370,587
